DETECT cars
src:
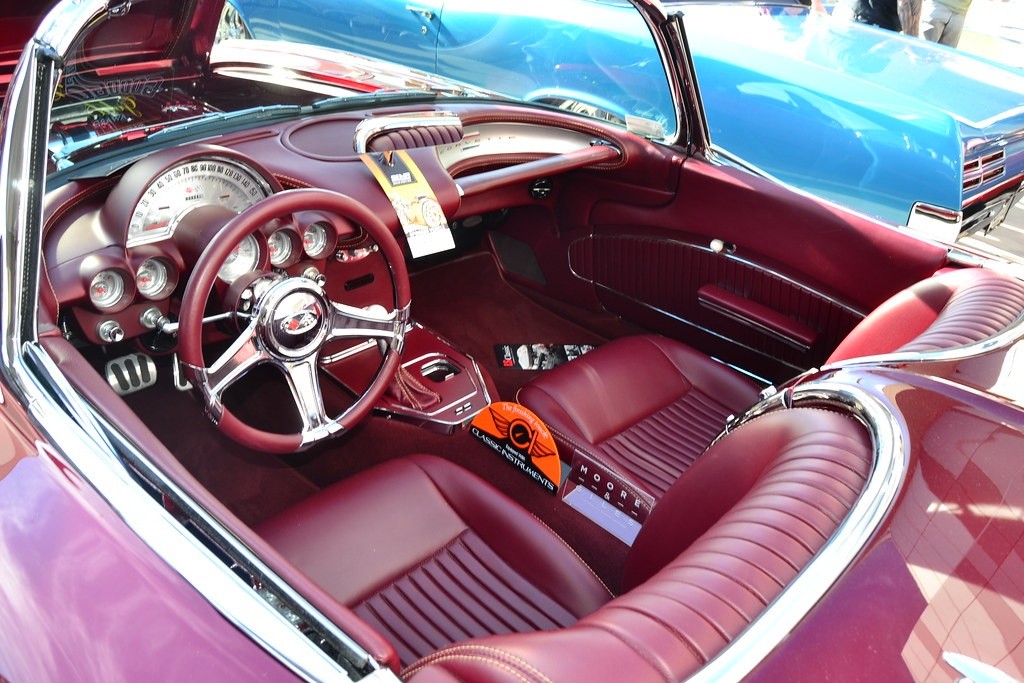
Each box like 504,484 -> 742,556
0,1 -> 1024,683
216,0 -> 1024,248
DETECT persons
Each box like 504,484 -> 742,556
752,0 -> 971,49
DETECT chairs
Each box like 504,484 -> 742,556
246,381 -> 907,683
516,266 -> 1024,508
